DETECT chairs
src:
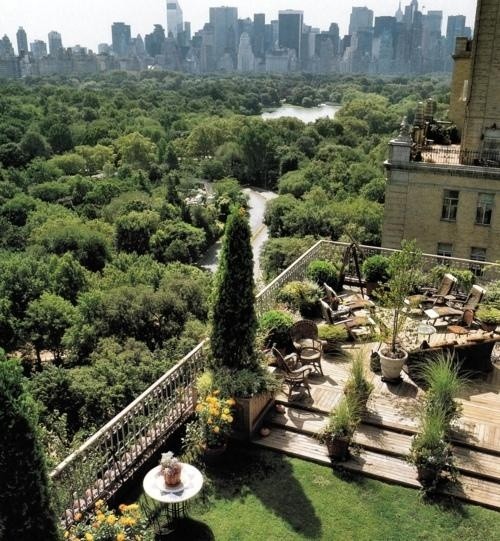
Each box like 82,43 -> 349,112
141,502 -> 176,541
270,320 -> 325,402
404,273 -> 486,344
319,281 -> 377,343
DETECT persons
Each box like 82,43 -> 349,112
330,295 -> 358,318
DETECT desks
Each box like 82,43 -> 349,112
141,462 -> 204,525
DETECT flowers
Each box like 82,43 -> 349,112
194,389 -> 235,447
62,498 -> 141,541
154,451 -> 182,479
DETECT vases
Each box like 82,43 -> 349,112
204,447 -> 228,457
164,471 -> 181,487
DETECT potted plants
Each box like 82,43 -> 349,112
310,351 -> 374,459
376,237 -> 425,382
397,347 -> 485,494
475,305 -> 500,333
